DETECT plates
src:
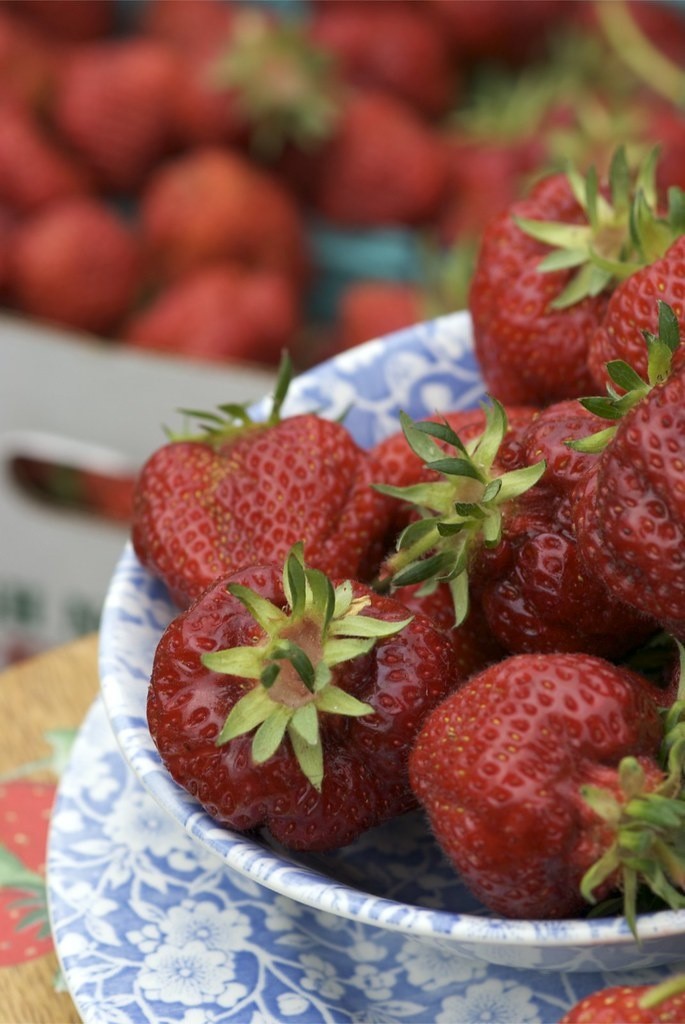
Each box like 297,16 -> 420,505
45,706 -> 685,1024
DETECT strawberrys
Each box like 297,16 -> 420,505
0,0 -> 685,1024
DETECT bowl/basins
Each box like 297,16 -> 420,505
92,314 -> 685,978
